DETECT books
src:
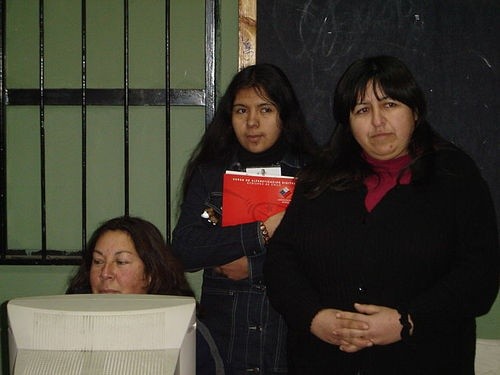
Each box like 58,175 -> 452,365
222,170 -> 296,231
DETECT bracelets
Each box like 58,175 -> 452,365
258,222 -> 269,242
395,308 -> 412,340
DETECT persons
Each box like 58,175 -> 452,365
265,50 -> 500,374
62,213 -> 227,375
169,61 -> 326,374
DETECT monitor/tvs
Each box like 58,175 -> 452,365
6,293 -> 197,375
